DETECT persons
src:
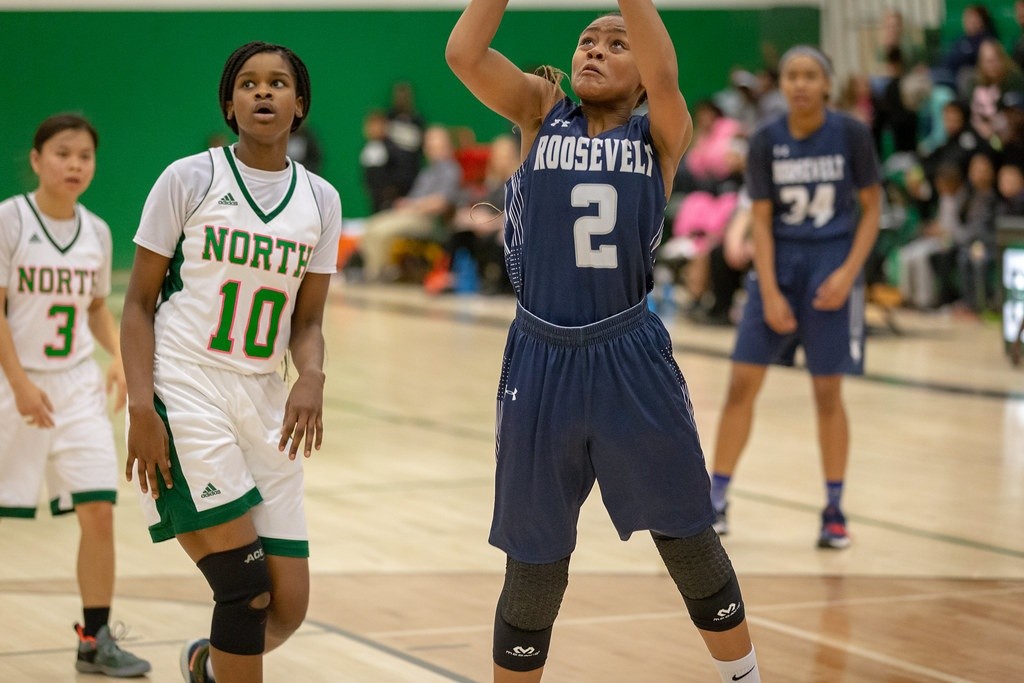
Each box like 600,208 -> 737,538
443,1 -> 760,683
710,45 -> 882,551
120,40 -> 343,683
0,115 -> 150,677
357,3 -> 1024,327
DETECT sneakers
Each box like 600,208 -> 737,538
711,500 -> 729,535
73,620 -> 151,677
817,504 -> 850,548
179,637 -> 216,683
